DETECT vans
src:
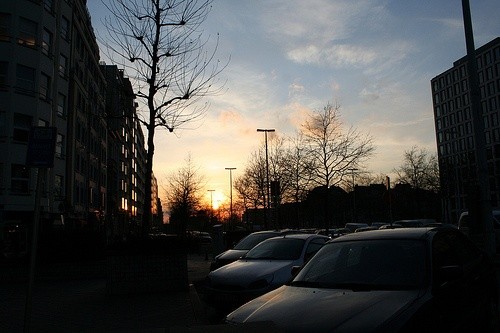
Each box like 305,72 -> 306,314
458,210 -> 500,254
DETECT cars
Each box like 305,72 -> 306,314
186,218 -> 443,246
222,228 -> 500,333
209,230 -> 305,272
207,233 -> 334,300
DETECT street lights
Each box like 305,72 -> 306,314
207,190 -> 216,226
225,167 -> 237,226
257,128 -> 275,230
348,168 -> 358,223
